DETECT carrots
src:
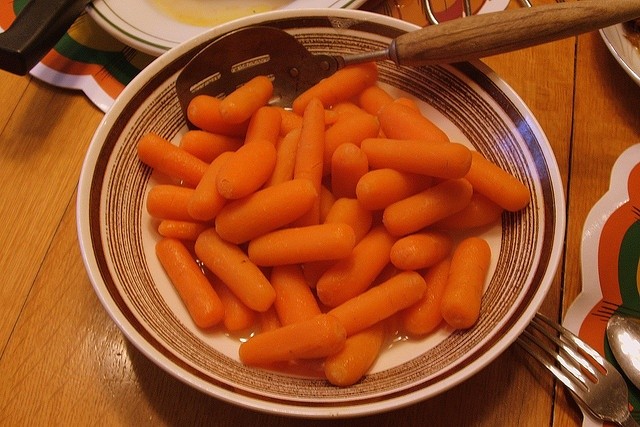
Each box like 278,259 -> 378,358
135,62 -> 532,385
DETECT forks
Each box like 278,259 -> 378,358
514,311 -> 638,426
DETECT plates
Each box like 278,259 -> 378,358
599,20 -> 639,85
85,0 -> 368,58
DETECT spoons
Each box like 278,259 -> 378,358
606,314 -> 639,393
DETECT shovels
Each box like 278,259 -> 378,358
175,0 -> 640,134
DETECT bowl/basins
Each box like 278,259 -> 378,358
74,7 -> 568,421
